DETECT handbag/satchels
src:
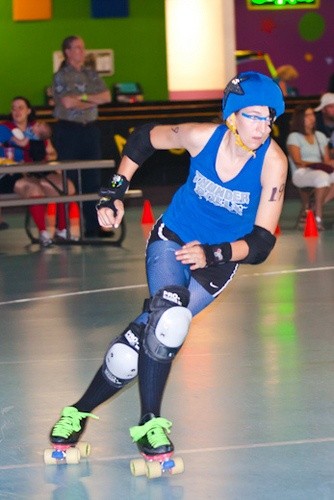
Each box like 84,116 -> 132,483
306,162 -> 334,175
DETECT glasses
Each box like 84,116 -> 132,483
237,110 -> 272,122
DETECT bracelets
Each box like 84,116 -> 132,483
81,94 -> 89,103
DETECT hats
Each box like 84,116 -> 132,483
221,69 -> 286,124
313,92 -> 334,112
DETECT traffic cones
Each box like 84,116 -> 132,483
139,199 -> 158,228
301,210 -> 320,239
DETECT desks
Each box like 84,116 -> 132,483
0,160 -> 126,246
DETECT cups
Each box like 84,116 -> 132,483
3,146 -> 14,160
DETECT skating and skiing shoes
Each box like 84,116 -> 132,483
129,412 -> 185,479
44,402 -> 99,465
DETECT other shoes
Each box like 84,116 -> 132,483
40,234 -> 52,246
298,212 -> 305,231
315,216 -> 325,232
56,231 -> 78,241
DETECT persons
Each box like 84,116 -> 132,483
44,70 -> 288,479
285,92 -> 334,231
0,35 -> 112,247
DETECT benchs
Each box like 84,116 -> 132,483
0,190 -> 144,207
